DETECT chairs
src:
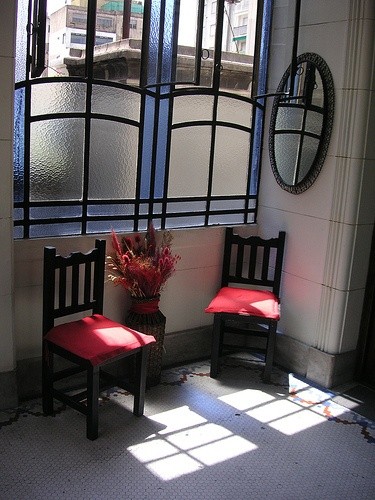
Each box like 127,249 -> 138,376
42,238 -> 157,442
208,226 -> 287,384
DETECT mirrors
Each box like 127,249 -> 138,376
267,51 -> 336,196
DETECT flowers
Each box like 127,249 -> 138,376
102,218 -> 182,299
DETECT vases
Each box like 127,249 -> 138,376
123,292 -> 168,388
